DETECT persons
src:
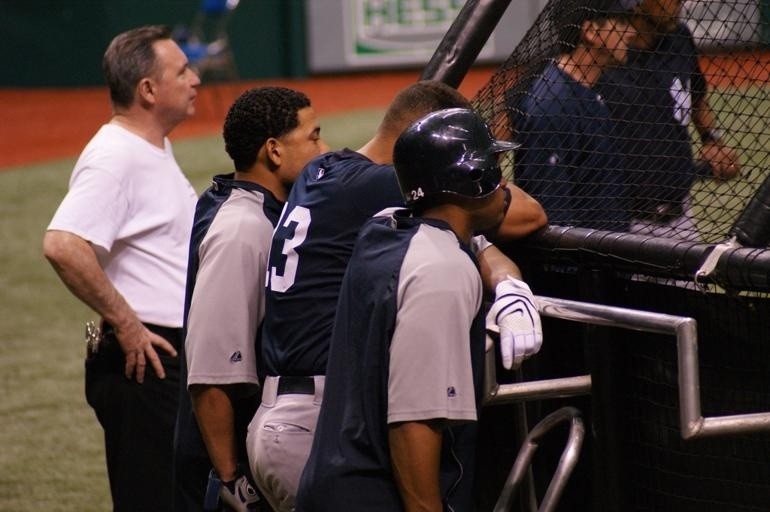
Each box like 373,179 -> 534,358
244,82 -> 549,512
293,107 -> 543,512
42,27 -> 201,512
598,0 -> 735,245
172,86 -> 323,512
514,0 -> 639,231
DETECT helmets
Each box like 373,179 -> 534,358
393,107 -> 521,205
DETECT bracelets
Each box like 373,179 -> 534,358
701,128 -> 723,142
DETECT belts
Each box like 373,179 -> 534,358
277,376 -> 314,395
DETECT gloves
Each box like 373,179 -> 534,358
496,281 -> 543,371
220,474 -> 261,512
